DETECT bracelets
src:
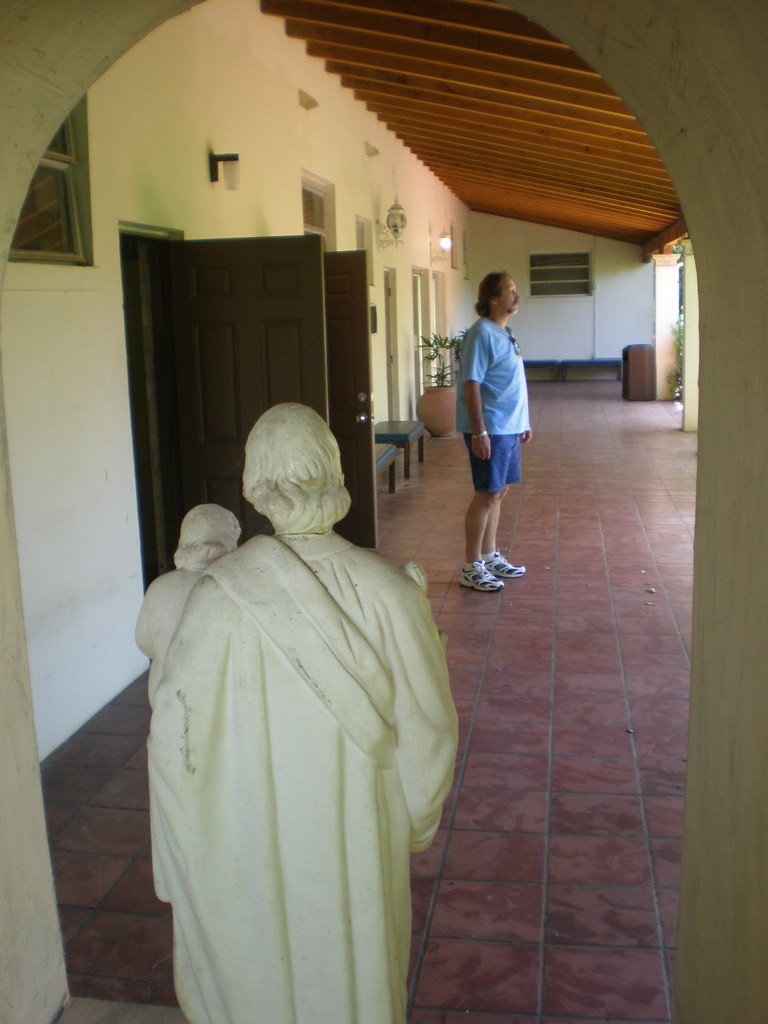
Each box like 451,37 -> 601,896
472,431 -> 487,439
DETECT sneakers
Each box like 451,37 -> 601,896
459,560 -> 505,592
484,547 -> 526,577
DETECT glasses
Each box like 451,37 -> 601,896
509,336 -> 520,355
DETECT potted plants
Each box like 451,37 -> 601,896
415,330 -> 463,440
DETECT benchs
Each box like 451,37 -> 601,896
522,359 -> 561,382
374,419 -> 426,481
375,442 -> 397,500
561,357 -> 624,381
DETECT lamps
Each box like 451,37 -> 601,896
375,193 -> 412,253
428,227 -> 456,266
207,149 -> 244,193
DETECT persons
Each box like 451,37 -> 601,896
455,272 -> 532,593
132,404 -> 458,1024
